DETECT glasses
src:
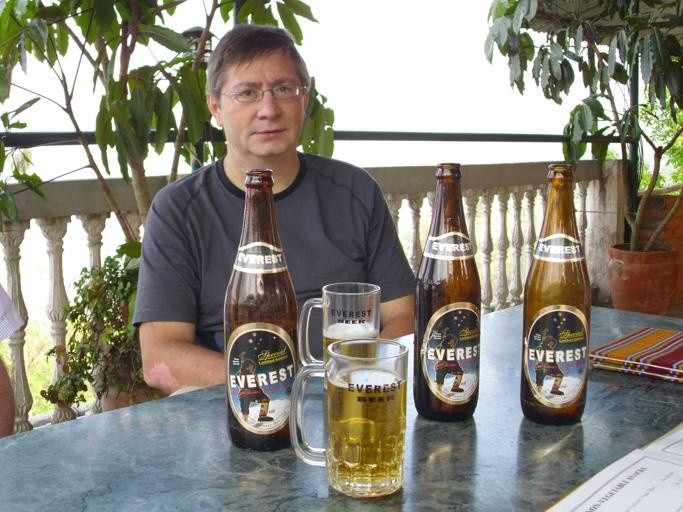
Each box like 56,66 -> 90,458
220,82 -> 307,104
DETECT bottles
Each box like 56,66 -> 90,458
221,167 -> 299,452
413,161 -> 482,422
519,161 -> 591,425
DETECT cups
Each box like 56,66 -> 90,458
296,281 -> 381,392
286,340 -> 410,499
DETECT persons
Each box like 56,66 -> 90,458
133,24 -> 416,397
0,284 -> 25,438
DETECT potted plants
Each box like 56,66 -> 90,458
0,0 -> 337,414
485,0 -> 683,318
